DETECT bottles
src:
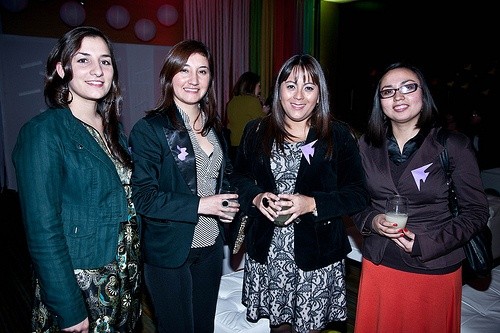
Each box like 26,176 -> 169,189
258,93 -> 273,118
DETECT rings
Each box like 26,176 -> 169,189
221,200 -> 231,208
262,197 -> 270,208
291,201 -> 294,207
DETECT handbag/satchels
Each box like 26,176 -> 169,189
225,207 -> 248,254
434,126 -> 493,292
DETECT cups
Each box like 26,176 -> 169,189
217,184 -> 241,223
271,189 -> 295,228
387,195 -> 409,232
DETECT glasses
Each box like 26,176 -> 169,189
378,83 -> 422,99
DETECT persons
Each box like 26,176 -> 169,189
127,40 -> 240,333
438,60 -> 500,166
352,62 -> 490,333
11,26 -> 145,333
232,55 -> 369,333
225,71 -> 272,180
